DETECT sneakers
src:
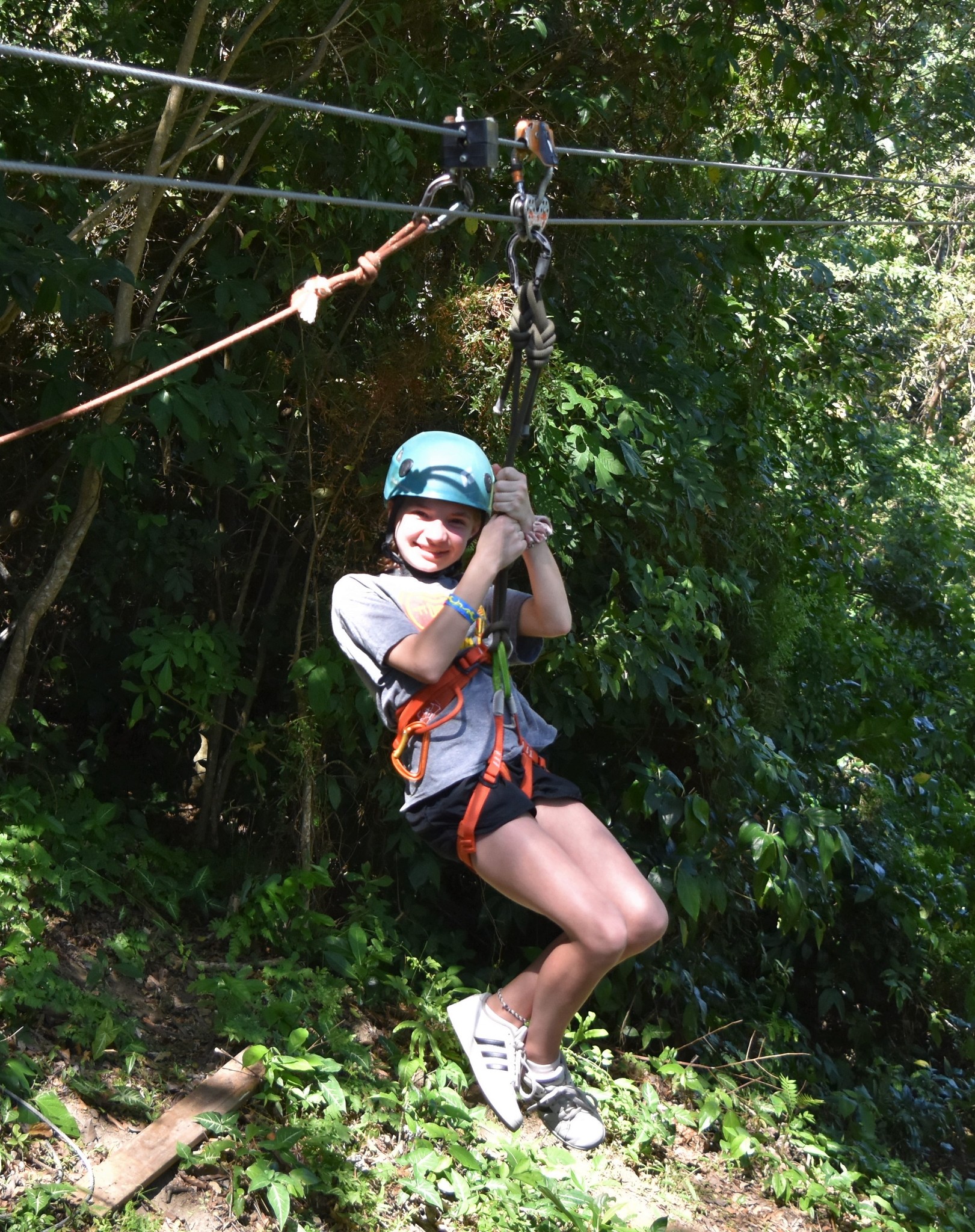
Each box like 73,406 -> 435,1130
447,993 -> 528,1131
522,1053 -> 606,1149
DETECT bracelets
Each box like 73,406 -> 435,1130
524,515 -> 552,549
444,594 -> 480,625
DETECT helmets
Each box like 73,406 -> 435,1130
383,431 -> 495,521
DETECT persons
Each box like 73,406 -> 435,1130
330,431 -> 670,1152
